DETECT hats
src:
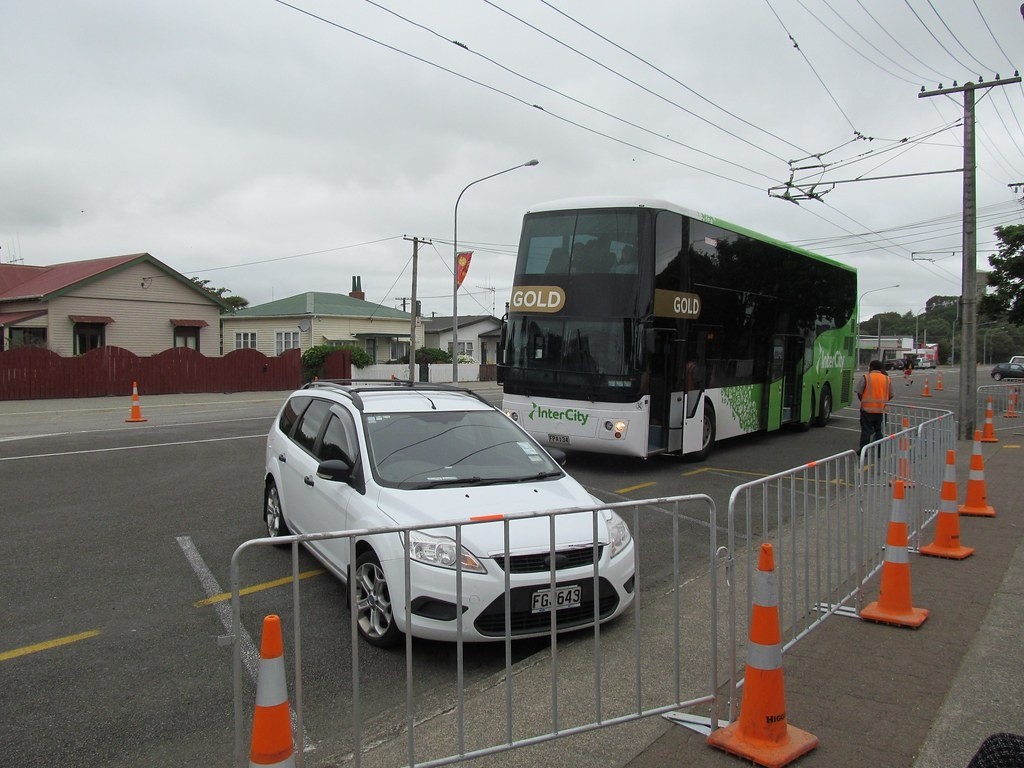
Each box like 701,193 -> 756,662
870,360 -> 882,368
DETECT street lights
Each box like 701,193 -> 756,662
453,159 -> 539,386
858,285 -> 900,372
916,303 -> 950,352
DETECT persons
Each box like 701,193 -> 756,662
561,237 -> 636,273
903,354 -> 914,386
854,360 -> 894,457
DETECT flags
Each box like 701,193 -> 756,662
456,252 -> 472,289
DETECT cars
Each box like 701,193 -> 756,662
883,357 -> 937,370
262,380 -> 636,645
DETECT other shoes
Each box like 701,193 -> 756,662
910,380 -> 913,384
855,448 -> 868,457
904,384 -> 909,386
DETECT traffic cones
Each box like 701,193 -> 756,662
126,382 -> 148,422
710,542 -> 820,767
918,449 -> 976,559
933,372 -> 944,391
241,612 -> 304,768
1000,385 -> 1020,418
958,430 -> 994,517
859,477 -> 931,630
978,395 -> 1002,443
1010,384 -> 1021,407
918,376 -> 932,397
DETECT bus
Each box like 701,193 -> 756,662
496,201 -> 858,462
